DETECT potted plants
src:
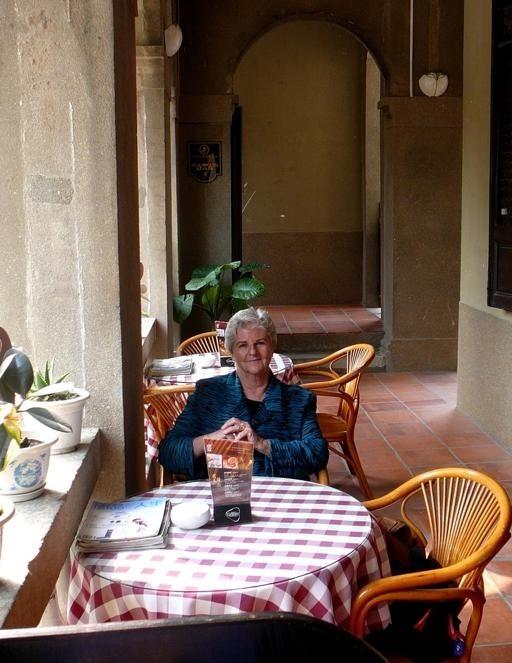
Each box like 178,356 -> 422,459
0,325 -> 91,561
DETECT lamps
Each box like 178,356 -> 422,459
163,22 -> 183,57
418,71 -> 448,97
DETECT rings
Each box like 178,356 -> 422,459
240,423 -> 245,430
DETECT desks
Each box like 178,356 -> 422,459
70,475 -> 372,614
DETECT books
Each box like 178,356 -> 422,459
146,355 -> 195,377
76,496 -> 175,552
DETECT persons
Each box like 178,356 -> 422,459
156,304 -> 330,483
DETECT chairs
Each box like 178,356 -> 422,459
144,327 -> 377,497
340,466 -> 512,663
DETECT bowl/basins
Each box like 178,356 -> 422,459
195,356 -> 216,368
169,502 -> 212,531
